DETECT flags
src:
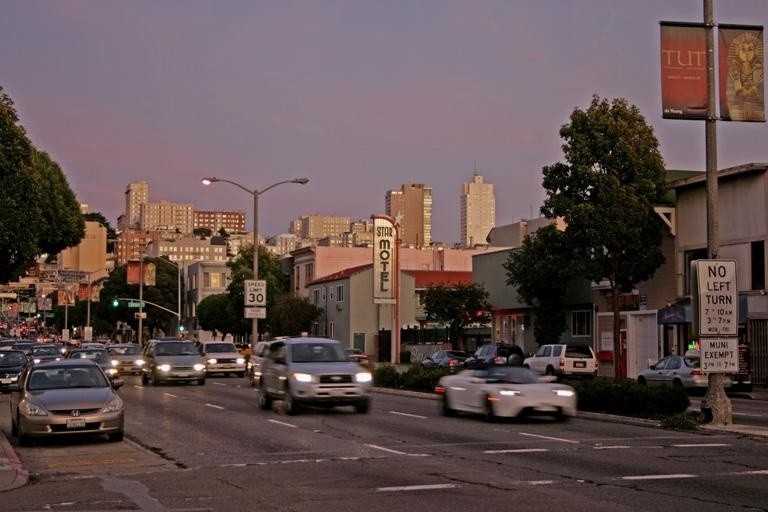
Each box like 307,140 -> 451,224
126,261 -> 140,284
78,284 -> 88,302
12,289 -> 77,314
716,24 -> 766,123
143,262 -> 156,286
91,283 -> 101,303
656,19 -> 711,121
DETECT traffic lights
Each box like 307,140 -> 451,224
112,298 -> 119,308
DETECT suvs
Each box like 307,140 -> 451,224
463,343 -> 523,370
141,337 -> 206,386
523,343 -> 598,377
256,337 -> 374,417
247,341 -> 270,387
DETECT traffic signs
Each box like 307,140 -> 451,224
134,312 -> 147,320
699,336 -> 741,375
127,302 -> 145,308
695,258 -> 739,337
243,280 -> 266,307
243,306 -> 268,318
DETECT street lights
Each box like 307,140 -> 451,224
86,267 -> 112,326
139,238 -> 175,343
6,279 -> 82,334
200,176 -> 309,353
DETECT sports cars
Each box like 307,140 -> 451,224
434,363 -> 578,424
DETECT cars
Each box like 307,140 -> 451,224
0,321 -> 142,392
199,340 -> 246,378
347,347 -> 369,368
6,359 -> 125,445
637,354 -> 733,392
420,350 -> 470,374
